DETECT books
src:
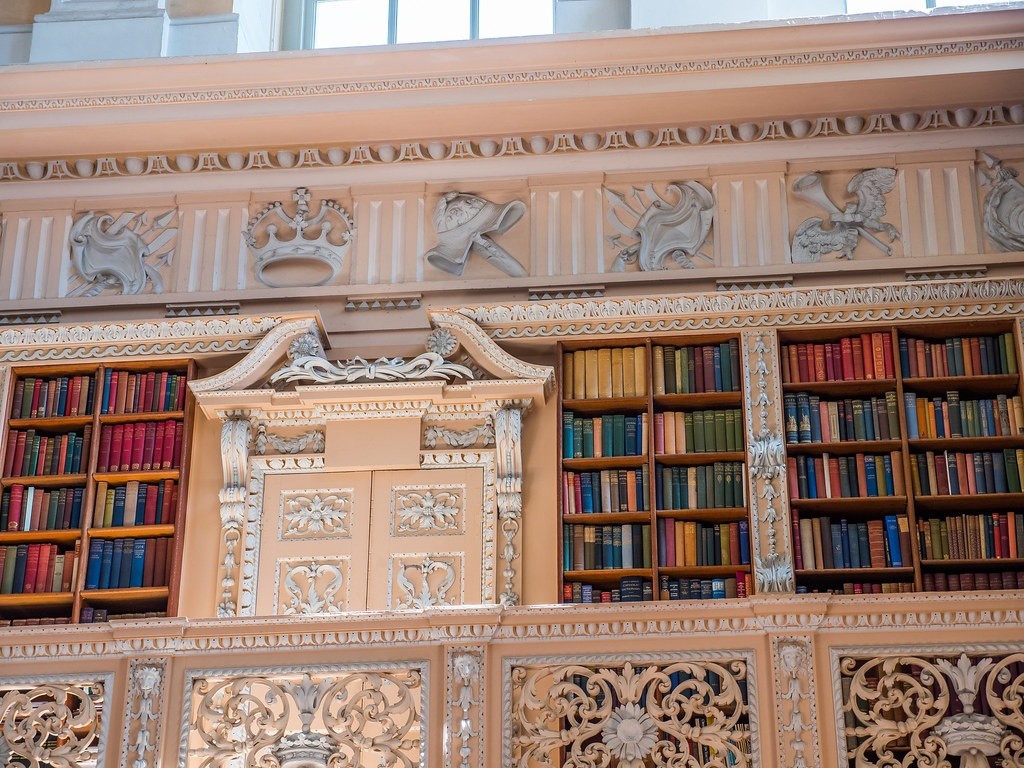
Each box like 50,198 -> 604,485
560,329 -> 1024,603
0,367 -> 187,628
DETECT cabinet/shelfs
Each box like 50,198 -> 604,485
0,317 -> 1024,768
